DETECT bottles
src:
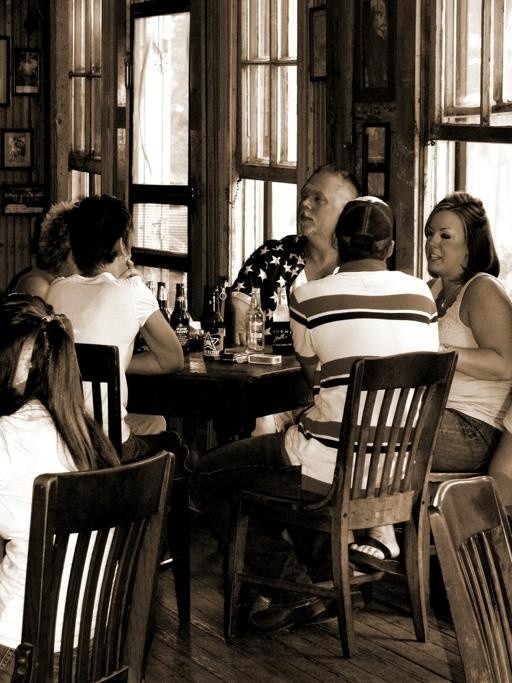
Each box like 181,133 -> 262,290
170,283 -> 190,357
272,286 -> 294,357
202,285 -> 225,363
158,282 -> 171,324
246,288 -> 265,350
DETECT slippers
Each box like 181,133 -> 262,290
347,537 -> 406,576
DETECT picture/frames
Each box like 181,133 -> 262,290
0,35 -> 47,217
309,6 -> 332,81
352,0 -> 397,202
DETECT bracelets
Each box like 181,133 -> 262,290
440,342 -> 450,352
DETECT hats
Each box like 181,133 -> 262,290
335,196 -> 394,251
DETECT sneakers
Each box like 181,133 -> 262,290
251,598 -> 325,634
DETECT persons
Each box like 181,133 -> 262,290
11,198 -> 83,303
42,192 -> 201,563
192,194 -> 440,631
215,159 -> 363,449
1,290 -> 125,683
345,189 -> 511,572
